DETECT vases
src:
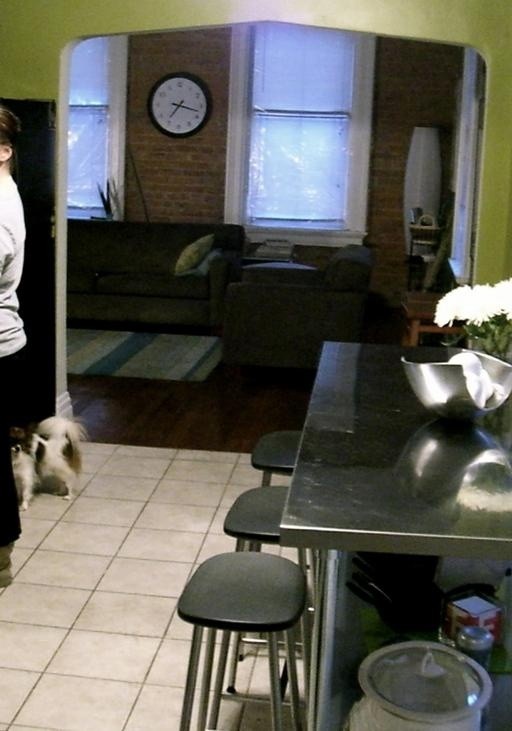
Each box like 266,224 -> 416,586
403,125 -> 443,258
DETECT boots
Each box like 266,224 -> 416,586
1,544 -> 13,586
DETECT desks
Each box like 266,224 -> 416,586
278,340 -> 512,731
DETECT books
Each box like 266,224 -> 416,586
255,239 -> 294,261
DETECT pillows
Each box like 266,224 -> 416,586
174,232 -> 215,274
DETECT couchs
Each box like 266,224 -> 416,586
67,219 -> 246,339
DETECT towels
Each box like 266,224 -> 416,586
442,347 -> 512,413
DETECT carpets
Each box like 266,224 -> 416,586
67,328 -> 226,385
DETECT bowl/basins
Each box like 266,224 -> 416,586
395,420 -> 512,516
399,347 -> 512,423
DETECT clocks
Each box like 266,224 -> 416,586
146,69 -> 213,138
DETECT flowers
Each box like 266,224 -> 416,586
433,275 -> 512,361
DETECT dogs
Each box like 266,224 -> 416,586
9,414 -> 90,511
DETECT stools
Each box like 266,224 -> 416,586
252,430 -> 305,553
400,292 -> 466,347
174,549 -> 308,730
220,486 -> 327,699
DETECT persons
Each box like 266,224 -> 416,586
1,104 -> 32,587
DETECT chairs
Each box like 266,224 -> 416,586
225,243 -> 372,369
